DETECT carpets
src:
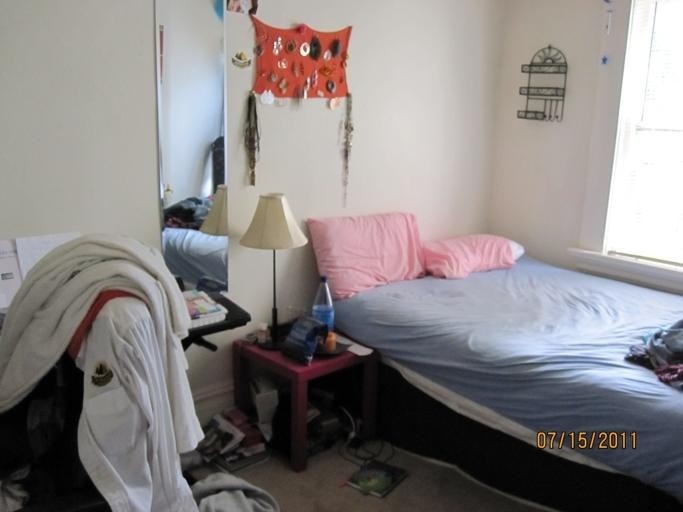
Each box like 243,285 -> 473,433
230,432 -> 548,512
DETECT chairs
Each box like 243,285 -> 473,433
0,351 -> 112,511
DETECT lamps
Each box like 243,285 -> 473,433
198,184 -> 228,235
240,194 -> 307,350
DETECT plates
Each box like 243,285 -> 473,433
312,341 -> 353,357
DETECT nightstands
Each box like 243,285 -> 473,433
233,321 -> 379,472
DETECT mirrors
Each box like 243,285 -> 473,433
157,0 -> 226,291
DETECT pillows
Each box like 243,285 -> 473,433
421,233 -> 525,279
308,212 -> 425,299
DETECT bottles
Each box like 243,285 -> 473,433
313,277 -> 334,333
162,184 -> 175,205
322,332 -> 338,354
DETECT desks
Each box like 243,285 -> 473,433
180,294 -> 251,353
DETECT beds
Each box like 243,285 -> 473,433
161,226 -> 228,292
332,258 -> 683,498
